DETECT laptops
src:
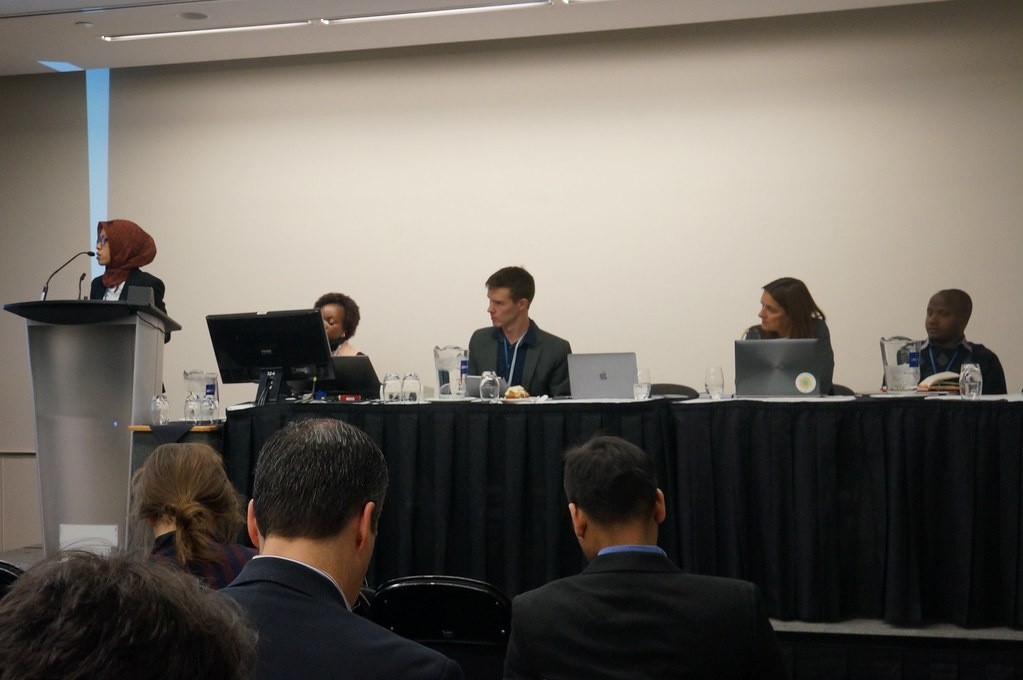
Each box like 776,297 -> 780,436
287,354 -> 381,400
567,352 -> 641,400
734,338 -> 829,398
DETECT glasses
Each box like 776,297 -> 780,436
97,237 -> 108,244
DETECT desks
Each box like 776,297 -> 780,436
669,392 -> 1023,630
218,391 -> 689,633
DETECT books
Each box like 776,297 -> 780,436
917,371 -> 961,391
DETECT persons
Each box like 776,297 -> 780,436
314,292 -> 381,400
467,266 -> 573,398
746,277 -> 835,396
203,418 -> 464,680
502,436 -> 786,680
90,219 -> 172,394
132,442 -> 259,593
0,546 -> 258,680
882,289 -> 1007,395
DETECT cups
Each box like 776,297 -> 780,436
184,395 -> 202,426
959,363 -> 983,400
383,373 -> 422,403
704,367 -> 724,400
632,369 -> 651,400
150,395 -> 170,425
479,375 -> 499,401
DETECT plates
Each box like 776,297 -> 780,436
500,398 -> 535,403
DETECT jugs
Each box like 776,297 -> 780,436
880,336 -> 921,394
182,370 -> 218,426
434,346 -> 470,398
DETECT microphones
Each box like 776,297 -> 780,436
40,252 -> 96,300
78,272 -> 86,300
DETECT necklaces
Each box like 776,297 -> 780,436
334,344 -> 341,356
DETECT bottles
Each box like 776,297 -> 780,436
202,384 -> 220,425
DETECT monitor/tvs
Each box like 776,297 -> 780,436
206,308 -> 337,405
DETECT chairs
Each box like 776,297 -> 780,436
648,383 -> 699,397
369,570 -> 512,680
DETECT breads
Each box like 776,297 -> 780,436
504,385 -> 531,399
